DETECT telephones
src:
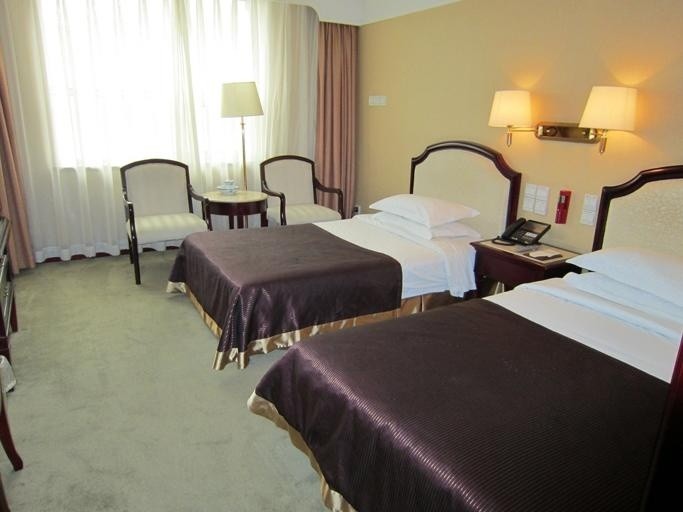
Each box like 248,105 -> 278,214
501,217 -> 551,246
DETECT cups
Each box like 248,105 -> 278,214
223,179 -> 236,190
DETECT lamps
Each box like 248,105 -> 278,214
219,81 -> 264,228
578,85 -> 639,156
487,90 -> 543,148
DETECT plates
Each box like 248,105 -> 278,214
215,185 -> 240,195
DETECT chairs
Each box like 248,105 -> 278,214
119,154 -> 346,285
